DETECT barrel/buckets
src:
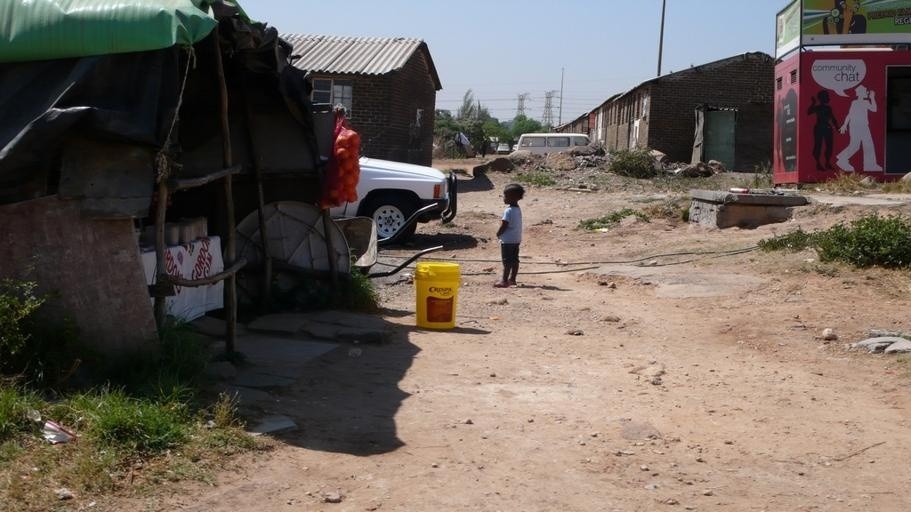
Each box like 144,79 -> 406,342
414,261 -> 462,331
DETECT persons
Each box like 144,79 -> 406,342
494,184 -> 525,288
824,0 -> 868,35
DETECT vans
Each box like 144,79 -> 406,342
517,133 -> 602,157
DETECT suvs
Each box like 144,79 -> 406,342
329,154 -> 458,247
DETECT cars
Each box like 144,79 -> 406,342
486,141 -> 517,155
